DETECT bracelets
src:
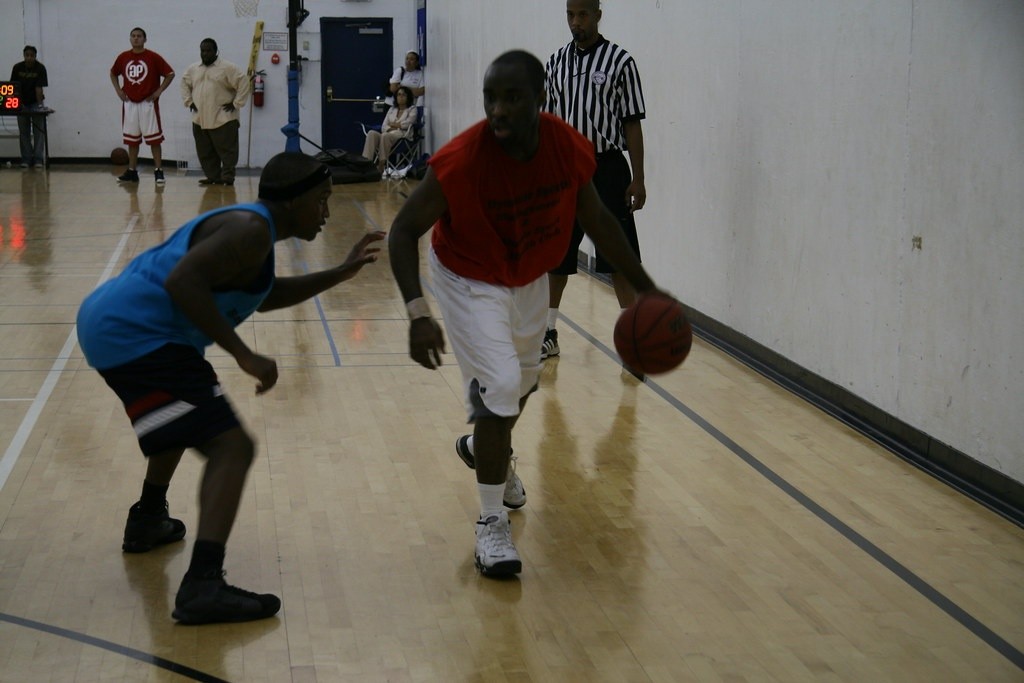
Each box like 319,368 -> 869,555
37,103 -> 43,109
405,297 -> 432,321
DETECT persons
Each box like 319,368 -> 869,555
9,46 -> 48,168
388,50 -> 660,576
77,151 -> 386,624
110,27 -> 175,182
362,52 -> 426,175
182,38 -> 250,185
537,0 -> 646,358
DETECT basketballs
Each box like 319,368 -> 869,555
111,147 -> 129,167
611,294 -> 693,375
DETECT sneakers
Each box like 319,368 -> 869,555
154,168 -> 165,183
537,326 -> 561,359
473,510 -> 522,577
170,570 -> 281,625
116,169 -> 139,183
455,434 -> 527,509
121,499 -> 186,552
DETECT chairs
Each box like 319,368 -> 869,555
360,106 -> 426,179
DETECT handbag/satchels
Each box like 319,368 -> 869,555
407,153 -> 430,180
383,66 -> 405,109
340,152 -> 376,172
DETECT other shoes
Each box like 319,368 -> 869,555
198,177 -> 224,184
33,162 -> 42,168
20,162 -> 29,167
224,179 -> 233,185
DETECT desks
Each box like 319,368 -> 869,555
0,108 -> 54,170
